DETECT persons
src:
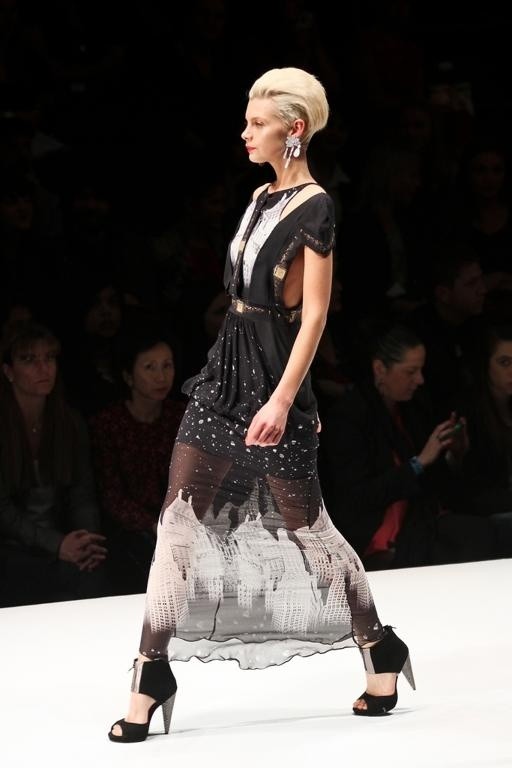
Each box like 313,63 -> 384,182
0,1 -> 512,608
104,63 -> 409,747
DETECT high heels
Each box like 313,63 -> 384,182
354,625 -> 416,716
110,655 -> 176,743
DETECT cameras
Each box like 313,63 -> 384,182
439,422 -> 464,440
